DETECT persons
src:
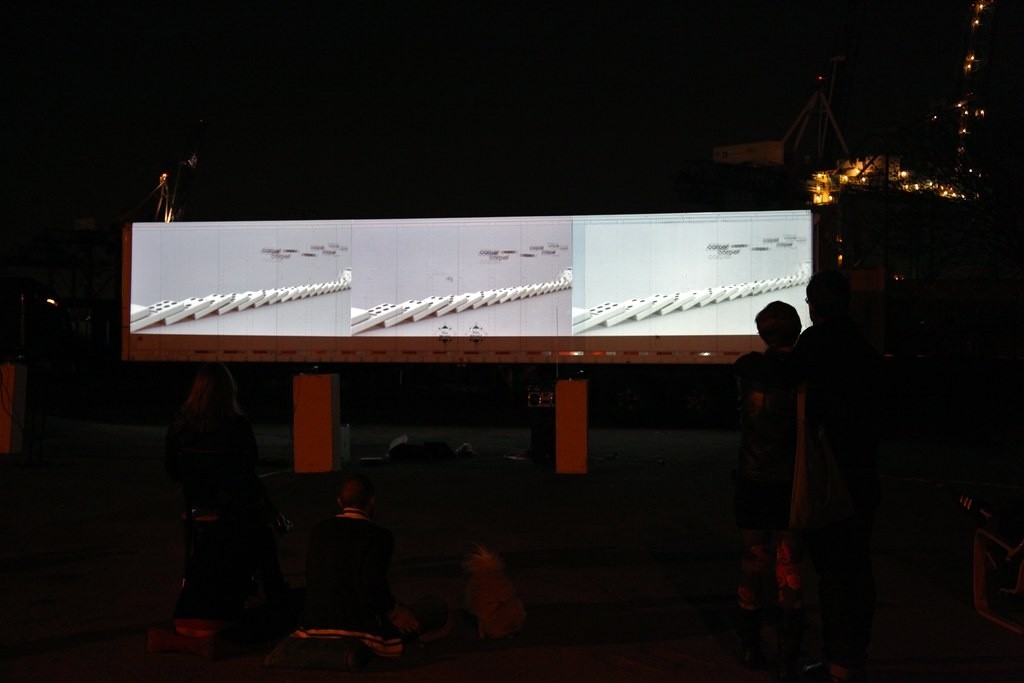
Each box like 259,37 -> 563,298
731,272 -> 892,683
300,462 -> 424,637
524,365 -> 569,463
163,361 -> 295,606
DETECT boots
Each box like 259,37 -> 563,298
774,601 -> 806,683
738,599 -> 769,669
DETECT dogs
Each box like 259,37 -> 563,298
463,542 -> 528,642
